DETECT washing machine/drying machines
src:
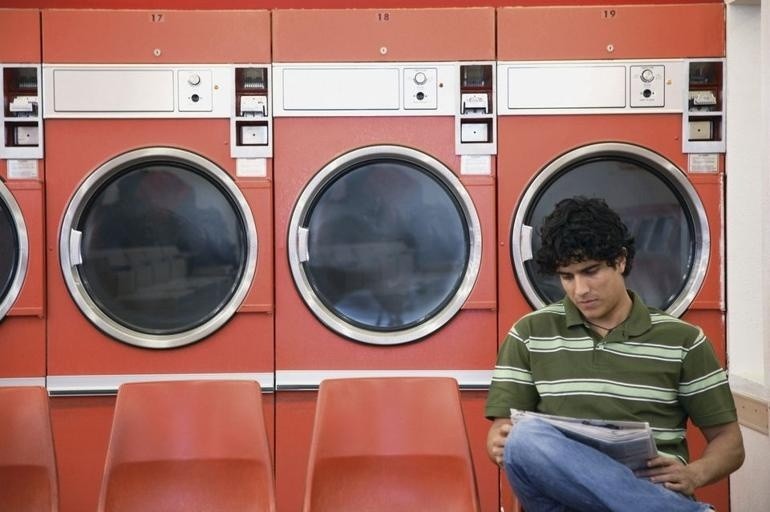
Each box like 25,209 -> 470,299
494,8 -> 733,512
269,6 -> 498,512
0,1 -> 45,384
38,4 -> 275,512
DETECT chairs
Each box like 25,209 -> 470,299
0,386 -> 58,512
97,380 -> 276,512
304,377 -> 481,512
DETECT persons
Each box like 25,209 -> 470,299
484,194 -> 746,512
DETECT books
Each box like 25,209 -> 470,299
503,398 -> 661,479
